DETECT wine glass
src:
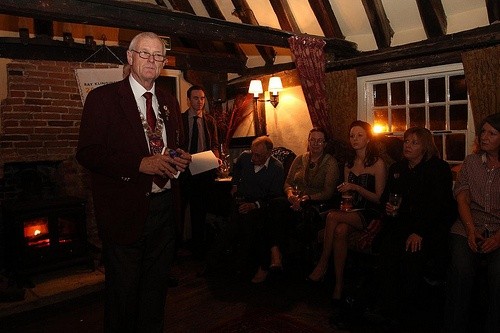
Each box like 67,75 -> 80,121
292,184 -> 304,210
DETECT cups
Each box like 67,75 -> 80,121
236,194 -> 248,214
342,191 -> 353,213
473,228 -> 490,253
388,193 -> 402,217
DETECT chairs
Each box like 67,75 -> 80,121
271,138 -> 398,304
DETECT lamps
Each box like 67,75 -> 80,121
248,77 -> 283,108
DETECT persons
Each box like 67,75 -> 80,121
252,127 -> 339,284
229,136 -> 285,251
77,31 -> 192,333
446,113 -> 500,333
305,120 -> 386,302
365,127 -> 457,317
173,86 -> 225,266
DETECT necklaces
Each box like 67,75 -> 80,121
136,105 -> 164,154
305,151 -> 327,194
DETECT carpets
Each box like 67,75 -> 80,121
208,310 -> 326,333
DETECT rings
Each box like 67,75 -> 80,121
343,189 -> 344,191
342,182 -> 344,185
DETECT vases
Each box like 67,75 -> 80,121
215,143 -> 230,178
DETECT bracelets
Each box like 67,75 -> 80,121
306,194 -> 311,200
285,187 -> 294,192
463,223 -> 475,231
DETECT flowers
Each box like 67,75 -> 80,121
208,95 -> 255,147
